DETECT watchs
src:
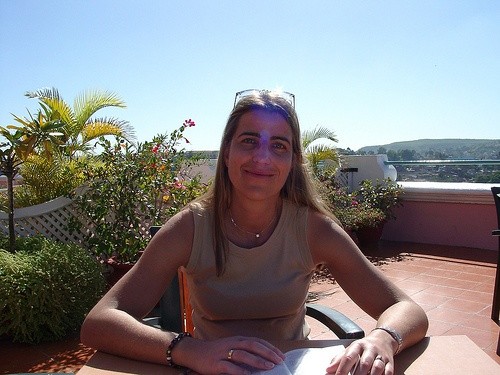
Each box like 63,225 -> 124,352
371,327 -> 402,356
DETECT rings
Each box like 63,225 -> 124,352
376,355 -> 385,363
228,350 -> 234,360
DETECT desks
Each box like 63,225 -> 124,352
75,333 -> 500,375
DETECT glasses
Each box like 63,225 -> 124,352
233,88 -> 295,113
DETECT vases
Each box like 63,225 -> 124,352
107,250 -> 144,279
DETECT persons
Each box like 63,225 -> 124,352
80,88 -> 429,375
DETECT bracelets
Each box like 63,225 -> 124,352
166,332 -> 192,368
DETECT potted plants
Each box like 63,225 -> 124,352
354,175 -> 407,249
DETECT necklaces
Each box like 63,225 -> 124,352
228,208 -> 278,237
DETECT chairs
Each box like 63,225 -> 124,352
149,226 -> 366,340
